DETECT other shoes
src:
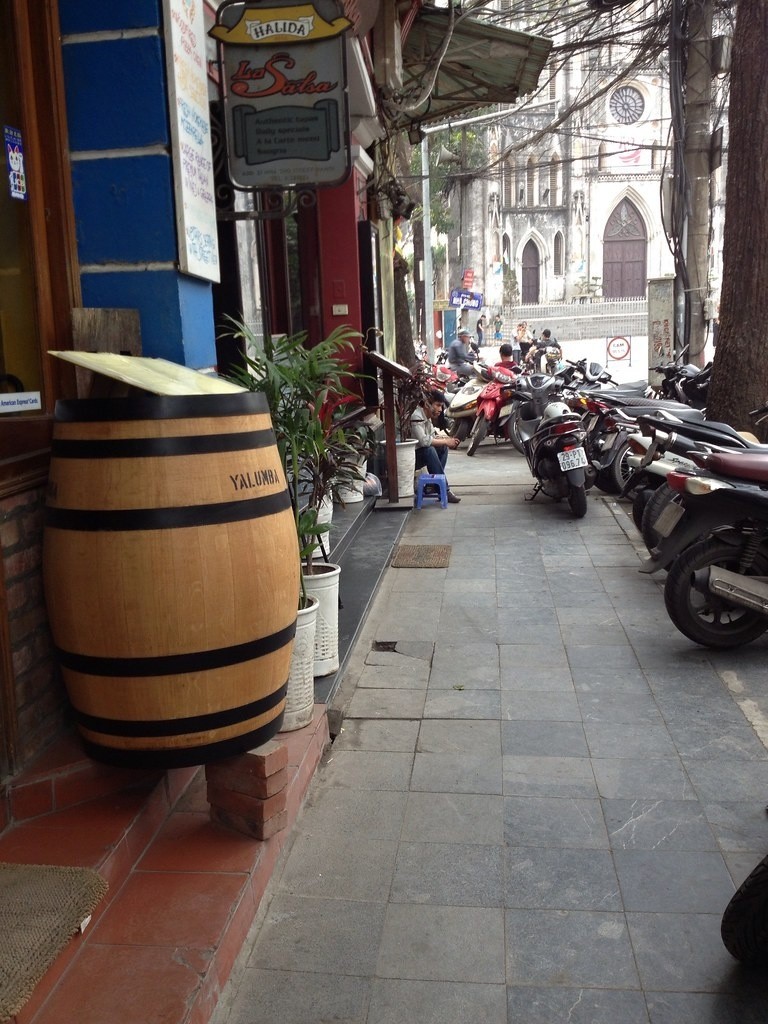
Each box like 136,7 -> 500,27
438,490 -> 461,503
424,484 -> 435,494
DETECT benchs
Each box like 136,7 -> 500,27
571,295 -> 593,303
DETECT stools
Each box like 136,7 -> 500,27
416,474 -> 448,510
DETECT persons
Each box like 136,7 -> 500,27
492,315 -> 504,346
494,344 -> 520,369
477,315 -> 486,346
448,330 -> 478,377
406,390 -> 461,503
510,322 -> 562,371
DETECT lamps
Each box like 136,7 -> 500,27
407,117 -> 427,144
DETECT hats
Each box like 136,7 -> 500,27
457,328 -> 473,337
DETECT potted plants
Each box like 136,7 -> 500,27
210,308 -> 379,735
378,362 -> 449,497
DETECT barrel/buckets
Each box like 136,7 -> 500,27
41,391 -> 300,769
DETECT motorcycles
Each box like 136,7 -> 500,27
564,344 -> 768,647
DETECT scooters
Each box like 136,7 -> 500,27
423,341 -> 620,518
